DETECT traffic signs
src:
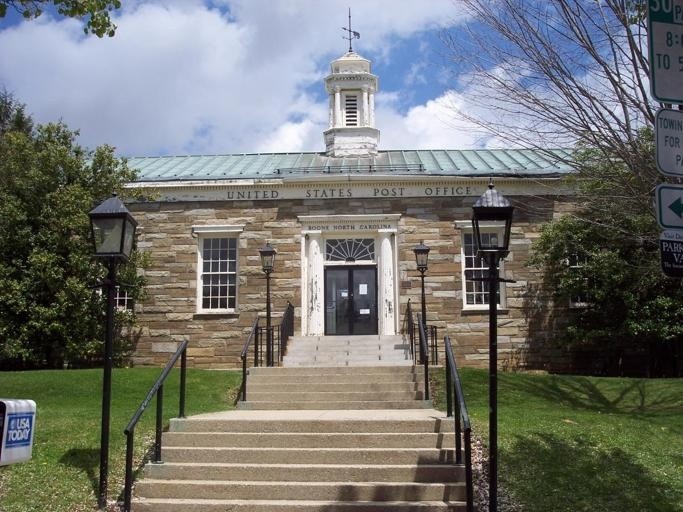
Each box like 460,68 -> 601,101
644,0 -> 683,279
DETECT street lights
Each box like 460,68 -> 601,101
413,240 -> 430,365
470,176 -> 515,511
87,188 -> 139,510
258,242 -> 277,366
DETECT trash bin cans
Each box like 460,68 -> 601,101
0,398 -> 37,467
